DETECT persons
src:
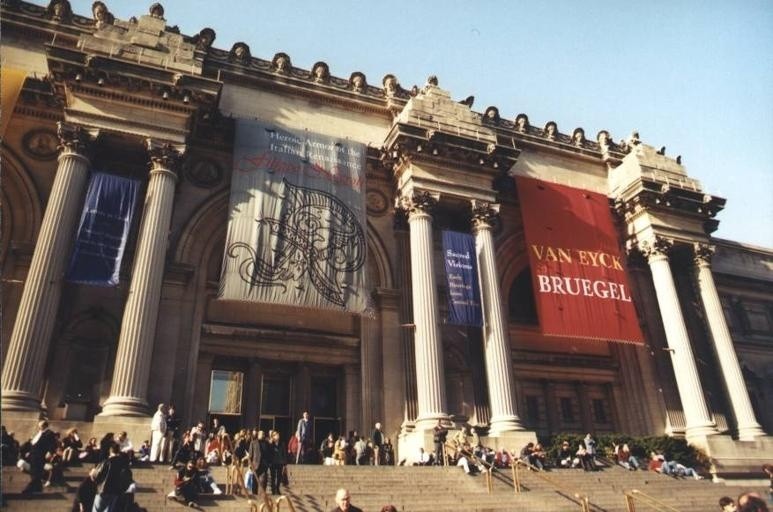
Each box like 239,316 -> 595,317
287,412 -> 397,512
1,404 -> 289,512
414,419 -> 549,476
556,433 -> 704,479
718,463 -> 773,512
